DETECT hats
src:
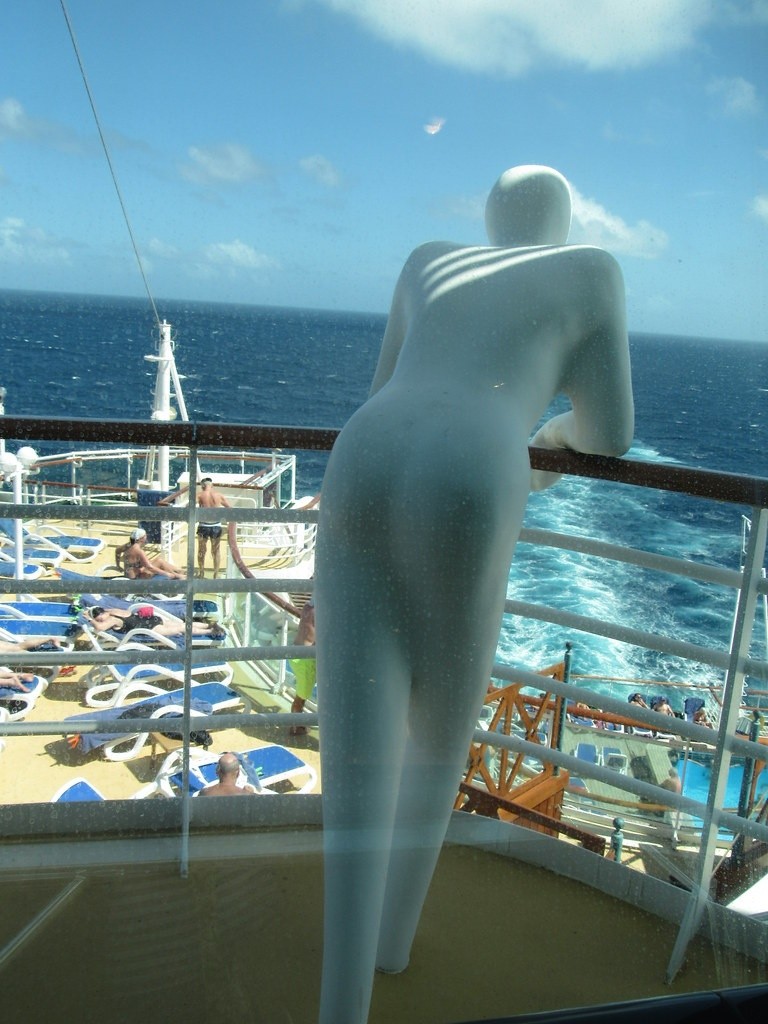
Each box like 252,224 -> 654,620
130,528 -> 147,540
91,606 -> 104,618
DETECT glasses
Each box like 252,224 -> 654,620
660,699 -> 665,701
638,697 -> 642,699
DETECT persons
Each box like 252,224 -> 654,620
288,603 -> 318,735
631,693 -> 650,710
116,529 -> 188,580
0,670 -> 36,693
576,702 -> 591,722
82,606 -> 227,637
196,478 -> 235,579
315,165 -> 634,1024
0,636 -> 60,653
693,707 -> 713,728
198,753 -> 255,796
651,696 -> 675,718
659,767 -> 681,794
531,693 -> 546,710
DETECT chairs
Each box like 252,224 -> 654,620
476,692 -> 710,818
1,517 -> 319,804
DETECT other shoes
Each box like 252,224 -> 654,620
288,725 -> 309,736
